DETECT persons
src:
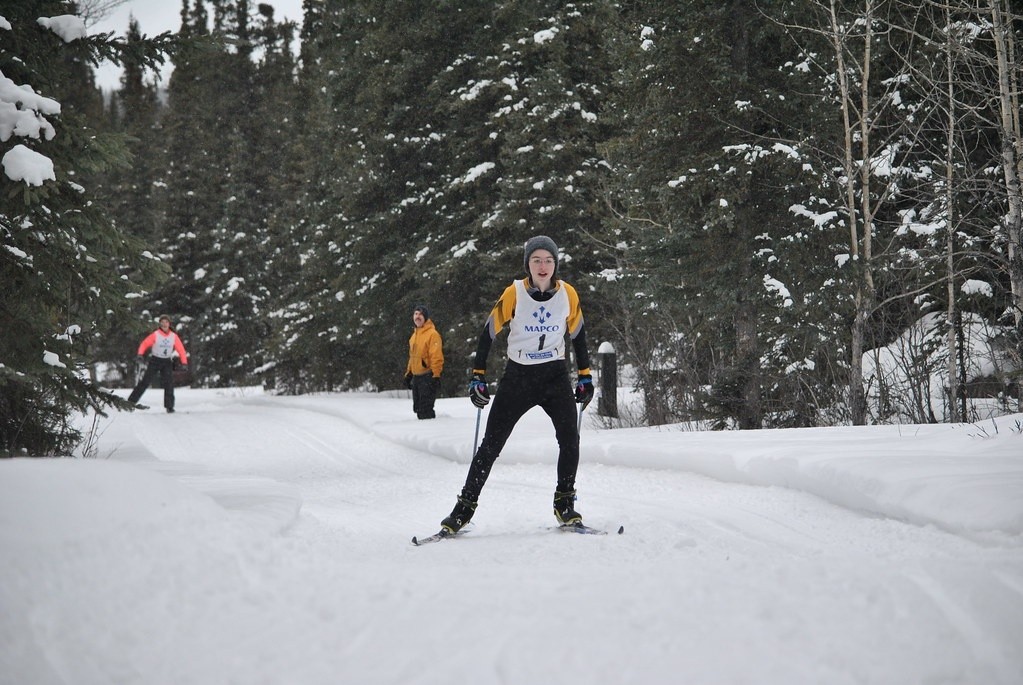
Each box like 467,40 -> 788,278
403,305 -> 444,419
441,236 -> 594,533
128,314 -> 187,413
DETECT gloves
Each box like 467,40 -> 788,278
469,376 -> 491,409
402,377 -> 412,389
574,374 -> 595,411
432,377 -> 441,388
181,365 -> 188,370
138,355 -> 144,361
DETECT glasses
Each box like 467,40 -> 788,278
527,258 -> 555,266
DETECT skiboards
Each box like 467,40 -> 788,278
411,524 -> 625,546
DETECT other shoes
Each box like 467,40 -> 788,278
166,408 -> 176,413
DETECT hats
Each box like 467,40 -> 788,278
159,314 -> 172,325
523,235 -> 559,276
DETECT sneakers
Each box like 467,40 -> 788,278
553,491 -> 583,525
441,495 -> 478,534
